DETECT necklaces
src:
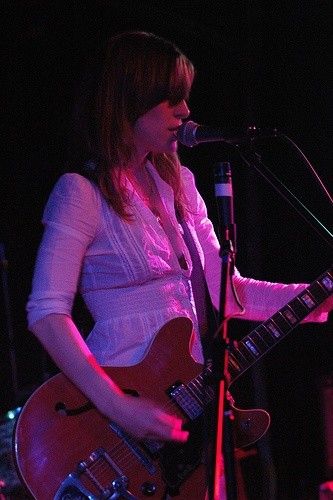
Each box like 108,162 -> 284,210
121,159 -> 164,228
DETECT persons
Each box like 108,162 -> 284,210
24,29 -> 333,499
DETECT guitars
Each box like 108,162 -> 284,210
10,265 -> 333,500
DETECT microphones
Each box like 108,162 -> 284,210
211,160 -> 236,243
174,120 -> 276,149
